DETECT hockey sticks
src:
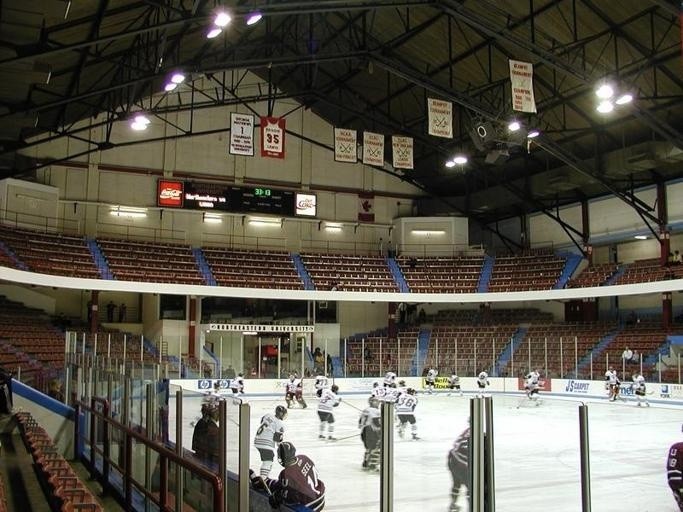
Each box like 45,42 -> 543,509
609,388 -> 626,403
327,434 -> 362,443
637,391 -> 654,394
252,476 -> 273,496
517,394 -> 528,409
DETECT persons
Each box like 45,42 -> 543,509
625,311 -> 636,329
671,249 -> 681,267
328,280 -> 344,291
54,310 -> 66,334
104,299 -> 116,323
377,237 -> 383,257
418,307 -> 427,325
387,239 -> 392,258
86,299 -> 92,322
484,301 -> 492,313
117,302 -> 127,323
397,302 -> 408,326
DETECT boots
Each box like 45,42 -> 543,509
449,504 -> 461,509
319,435 -> 336,440
361,462 -> 379,475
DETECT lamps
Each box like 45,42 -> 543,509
164,74 -> 185,92
110,210 -> 146,217
205,6 -> 263,39
508,121 -> 540,140
127,114 -> 152,134
445,153 -> 469,168
203,213 -> 223,222
594,79 -> 634,114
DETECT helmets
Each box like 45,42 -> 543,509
276,405 -> 287,417
277,442 -> 296,459
201,398 -> 219,422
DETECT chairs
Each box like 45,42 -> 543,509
2,297 -> 214,385
14,411 -> 103,512
0,225 -> 683,294
346,307 -> 682,384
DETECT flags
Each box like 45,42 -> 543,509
357,192 -> 375,223
